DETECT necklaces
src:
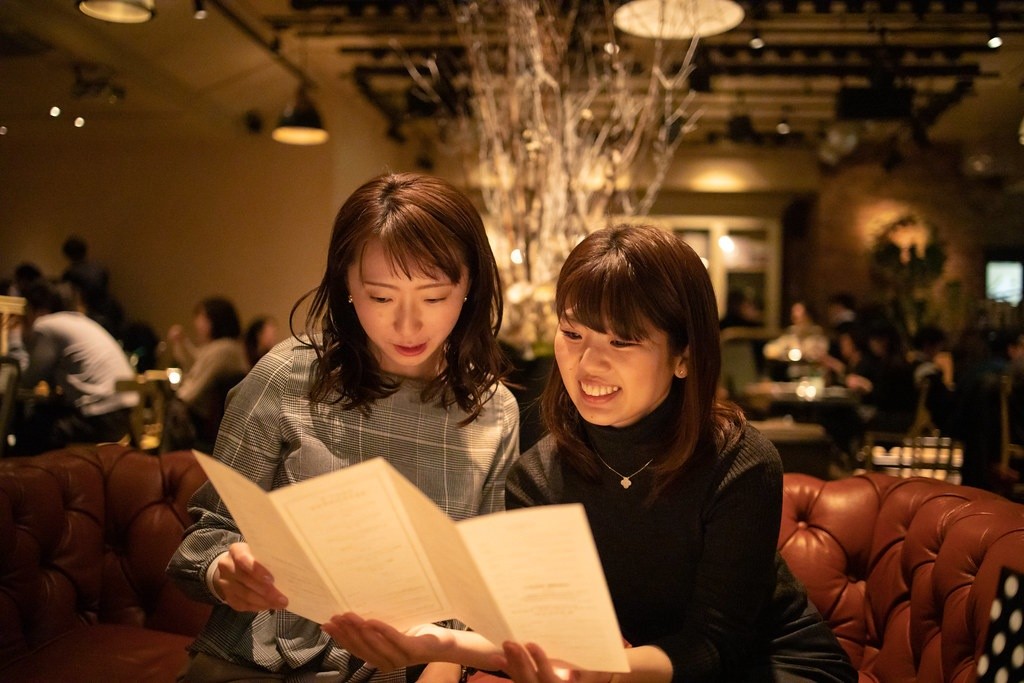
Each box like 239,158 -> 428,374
592,443 -> 654,489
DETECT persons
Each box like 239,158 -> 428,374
166,173 -> 519,683
318,224 -> 862,683
167,295 -> 279,422
0,233 -> 141,458
719,292 -> 1024,467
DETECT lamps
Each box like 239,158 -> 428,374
190,0 -> 207,20
612,0 -> 747,39
270,90 -> 330,143
77,0 -> 157,23
986,20 -> 1003,49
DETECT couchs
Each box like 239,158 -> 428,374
0,442 -> 1024,682
